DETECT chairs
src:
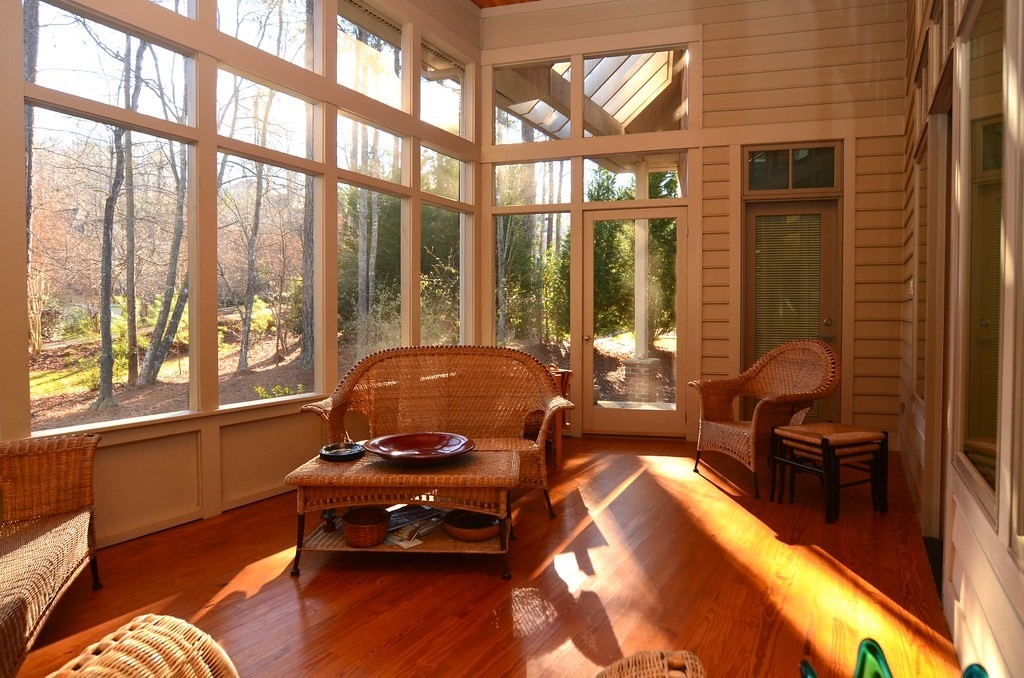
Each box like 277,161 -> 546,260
688,339 -> 841,500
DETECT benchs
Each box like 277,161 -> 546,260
0,434 -> 101,678
46,613 -> 705,678
298,345 -> 575,519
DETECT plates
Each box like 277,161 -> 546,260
318,442 -> 364,462
364,431 -> 476,466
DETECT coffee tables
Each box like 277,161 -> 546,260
284,452 -> 521,580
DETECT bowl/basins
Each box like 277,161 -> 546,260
442,508 -> 501,540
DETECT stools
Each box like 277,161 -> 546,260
769,421 -> 889,525
